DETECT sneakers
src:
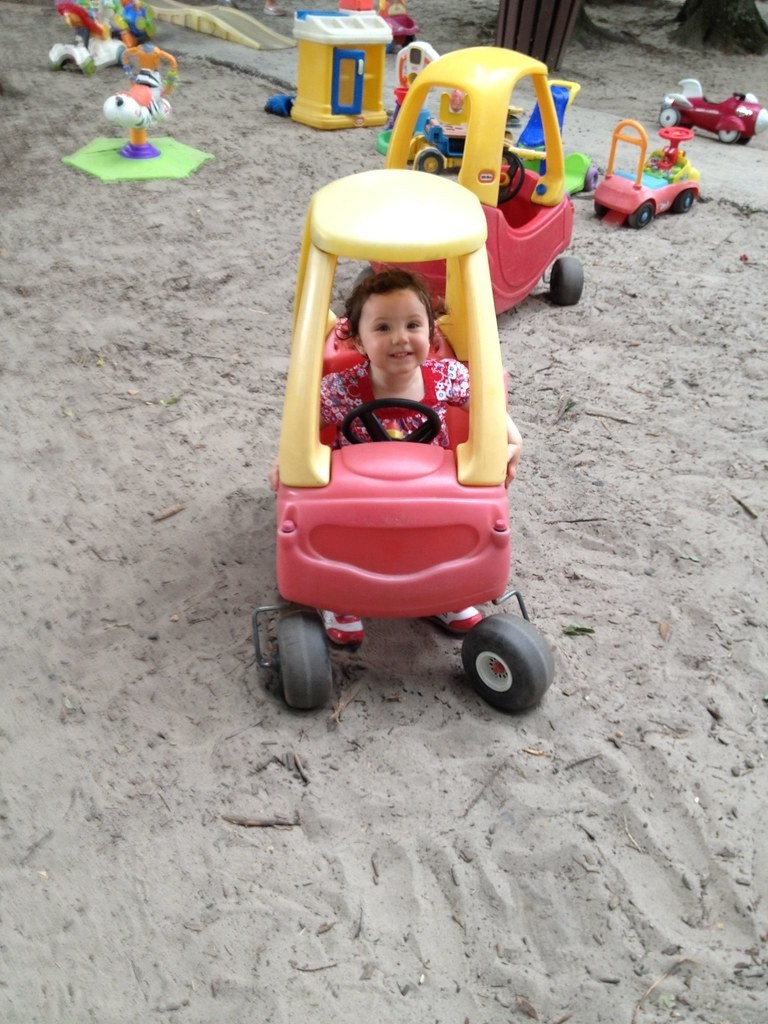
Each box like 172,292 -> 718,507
317,606 -> 364,646
432,604 -> 483,633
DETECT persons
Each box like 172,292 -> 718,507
270,269 -> 523,651
